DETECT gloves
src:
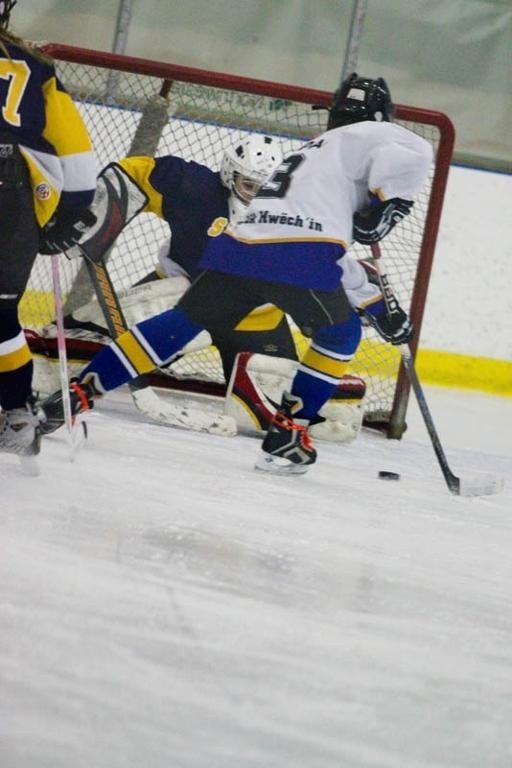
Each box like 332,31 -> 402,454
354,202 -> 411,244
361,299 -> 413,344
37,208 -> 97,256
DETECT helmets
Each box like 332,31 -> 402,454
221,134 -> 282,213
328,72 -> 392,122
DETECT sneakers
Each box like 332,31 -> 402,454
263,417 -> 317,464
2,381 -> 93,458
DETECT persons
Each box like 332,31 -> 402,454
34,69 -> 434,466
29,132 -> 367,437
1,0 -> 101,446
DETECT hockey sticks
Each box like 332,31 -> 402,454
372,243 -> 502,496
90,259 -> 237,437
51,258 -> 87,450
